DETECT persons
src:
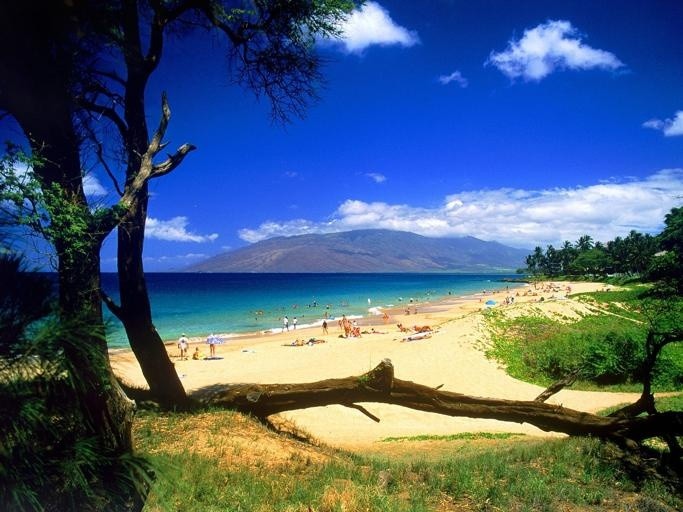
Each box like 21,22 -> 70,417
210,332 -> 216,357
177,332 -> 189,361
193,347 -> 199,361
282,280 -> 574,347
